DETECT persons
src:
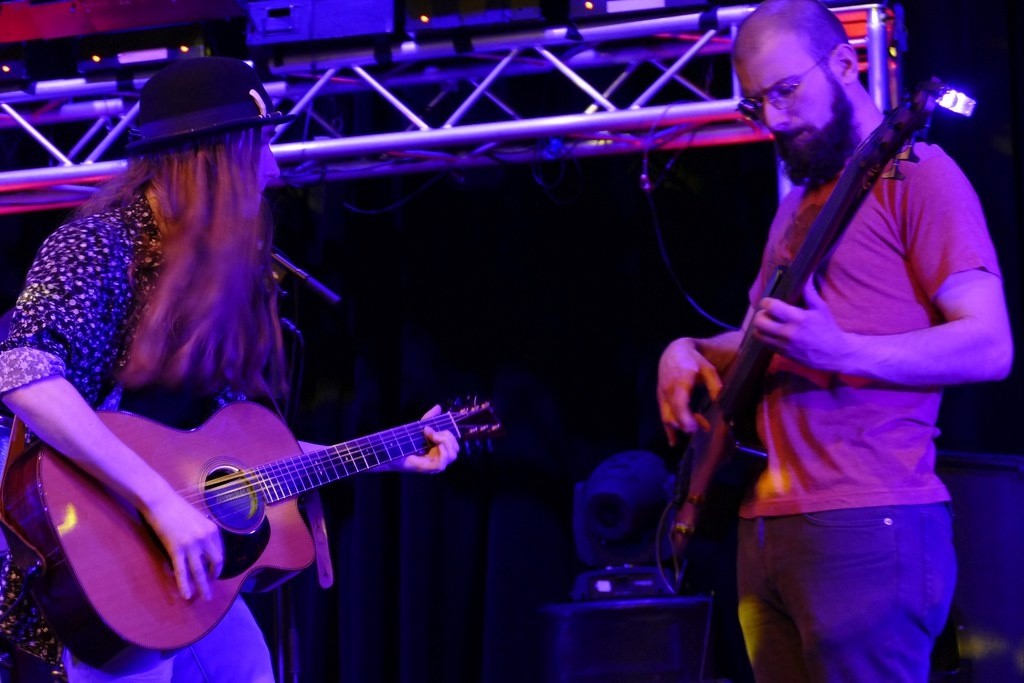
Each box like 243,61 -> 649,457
0,57 -> 459,683
655,0 -> 1013,683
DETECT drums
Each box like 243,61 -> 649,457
930,447 -> 1024,673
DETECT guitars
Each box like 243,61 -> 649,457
669,69 -> 948,565
0,391 -> 506,675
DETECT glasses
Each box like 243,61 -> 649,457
733,50 -> 835,117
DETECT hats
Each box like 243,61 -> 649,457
124,58 -> 296,149
